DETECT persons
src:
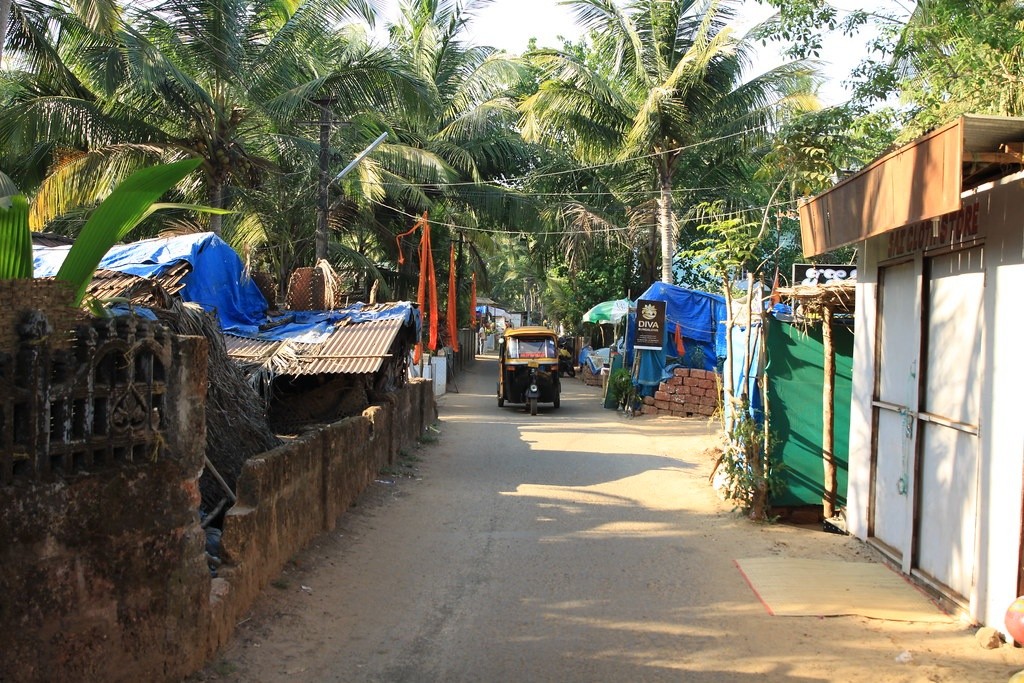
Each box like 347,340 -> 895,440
478,324 -> 487,355
609,330 -> 625,356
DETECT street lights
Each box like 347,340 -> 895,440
317,131 -> 387,265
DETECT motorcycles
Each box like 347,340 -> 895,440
496,326 -> 561,416
558,354 -> 576,378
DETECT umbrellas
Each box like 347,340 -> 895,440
582,299 -> 632,345
